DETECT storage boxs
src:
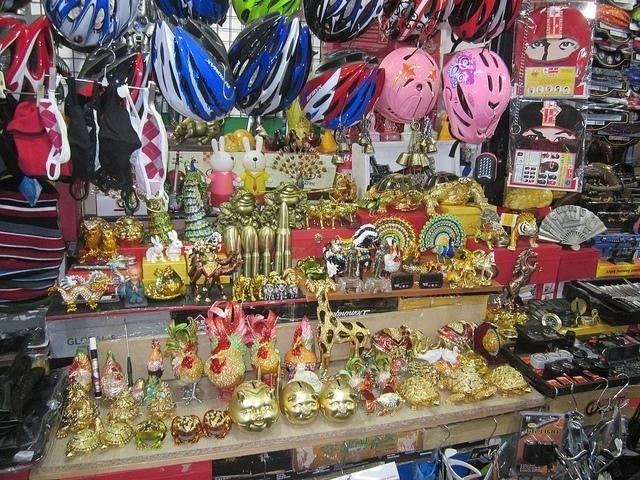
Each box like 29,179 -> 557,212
43,304 -> 547,480
466,234 -> 562,287
357,208 -> 436,257
558,247 -> 601,282
438,205 -> 482,235
291,227 -> 358,259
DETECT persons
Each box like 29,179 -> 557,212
125,267 -> 146,304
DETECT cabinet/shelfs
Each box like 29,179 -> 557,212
501,275 -> 640,479
1,1 -> 490,244
510,2 -> 639,277
44,207 -> 506,369
495,276 -> 640,431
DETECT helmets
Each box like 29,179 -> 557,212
228,14 -> 312,118
297,50 -> 387,134
43,0 -> 144,55
303,1 -> 385,46
0,12 -> 58,106
512,7 -> 592,86
381,0 -> 455,51
149,14 -> 236,125
233,1 -> 303,26
440,46 -> 514,146
448,0 -> 524,46
512,101 -> 584,172
152,0 -> 231,27
374,47 -> 443,124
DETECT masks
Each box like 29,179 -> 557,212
65,76 -> 141,211
139,89 -> 168,201
38,74 -> 70,180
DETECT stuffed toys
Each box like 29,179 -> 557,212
239,135 -> 274,207
206,135 -> 240,212
